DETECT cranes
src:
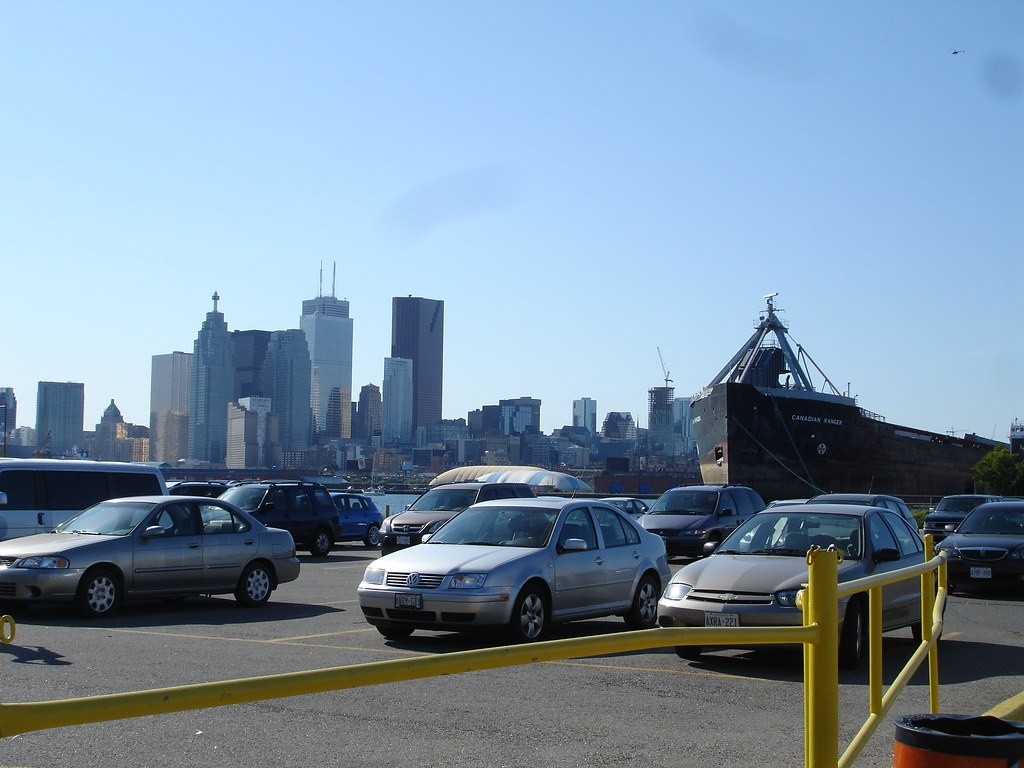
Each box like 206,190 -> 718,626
657,346 -> 673,387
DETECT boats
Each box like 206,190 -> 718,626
689,292 -> 1024,506
362,486 -> 385,496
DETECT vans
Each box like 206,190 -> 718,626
0,458 -> 172,543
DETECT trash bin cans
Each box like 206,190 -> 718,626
894,713 -> 1024,768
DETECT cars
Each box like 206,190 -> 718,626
740,495 -> 1024,597
297,492 -> 382,548
551,497 -> 650,538
356,498 -> 672,645
0,496 -> 300,619
656,503 -> 947,659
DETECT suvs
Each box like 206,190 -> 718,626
379,483 -> 548,557
165,481 -> 342,556
636,483 -> 767,558
